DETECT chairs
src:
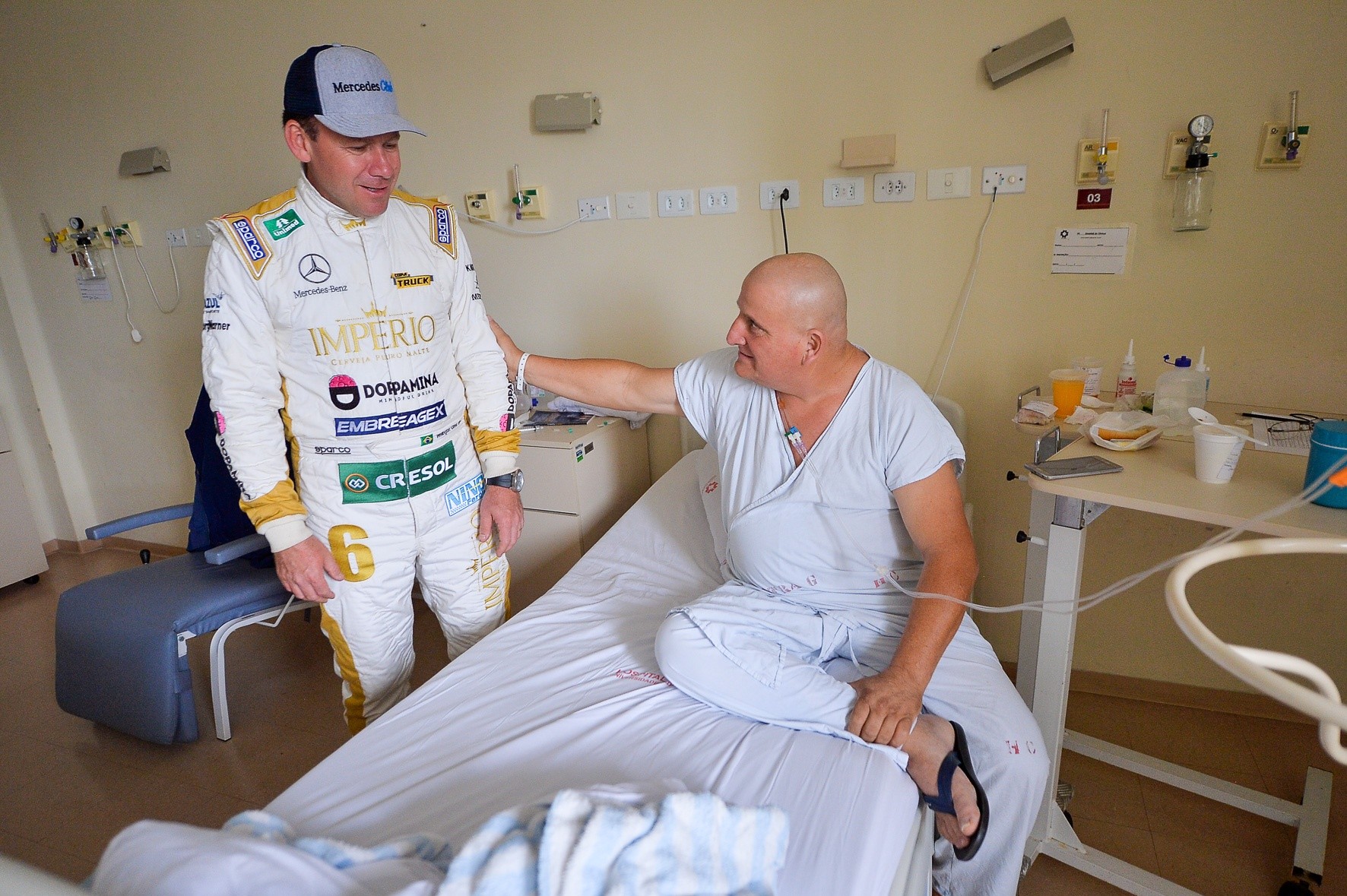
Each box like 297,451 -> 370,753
54,385 -> 319,746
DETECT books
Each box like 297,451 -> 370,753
523,410 -> 595,427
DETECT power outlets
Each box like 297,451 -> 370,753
657,189 -> 695,216
982,165 -> 1026,194
165,228 -> 188,247
873,172 -> 916,202
760,180 -> 799,210
577,196 -> 612,222
699,186 -> 737,215
823,177 -> 864,207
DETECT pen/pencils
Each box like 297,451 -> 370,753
1235,411 -> 1305,424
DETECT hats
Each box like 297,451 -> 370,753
284,43 -> 427,137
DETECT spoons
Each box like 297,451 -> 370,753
1081,395 -> 1114,408
1188,406 -> 1268,447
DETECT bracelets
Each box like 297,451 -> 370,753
513,353 -> 533,390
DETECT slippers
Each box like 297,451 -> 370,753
922,720 -> 989,861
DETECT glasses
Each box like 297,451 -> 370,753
1267,418 -> 1340,440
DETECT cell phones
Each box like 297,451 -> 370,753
1024,455 -> 1124,481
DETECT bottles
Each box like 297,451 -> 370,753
1195,345 -> 1210,401
77,236 -> 106,281
1151,354 -> 1207,431
1115,339 -> 1138,401
1172,153 -> 1214,232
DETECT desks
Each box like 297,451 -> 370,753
1016,392 -> 1347,896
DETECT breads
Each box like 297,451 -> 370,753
1098,425 -> 1155,441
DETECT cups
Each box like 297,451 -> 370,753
1075,357 -> 1104,399
1304,421 -> 1347,508
1049,369 -> 1090,419
1192,424 -> 1249,485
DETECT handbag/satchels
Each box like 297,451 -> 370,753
1078,410 -> 1178,451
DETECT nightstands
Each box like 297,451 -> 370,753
507,410 -> 651,606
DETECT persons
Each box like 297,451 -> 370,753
200,44 -> 525,738
487,252 -> 1049,896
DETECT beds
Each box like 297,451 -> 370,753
1,396 -> 968,896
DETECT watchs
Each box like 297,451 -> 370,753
482,468 -> 524,493
